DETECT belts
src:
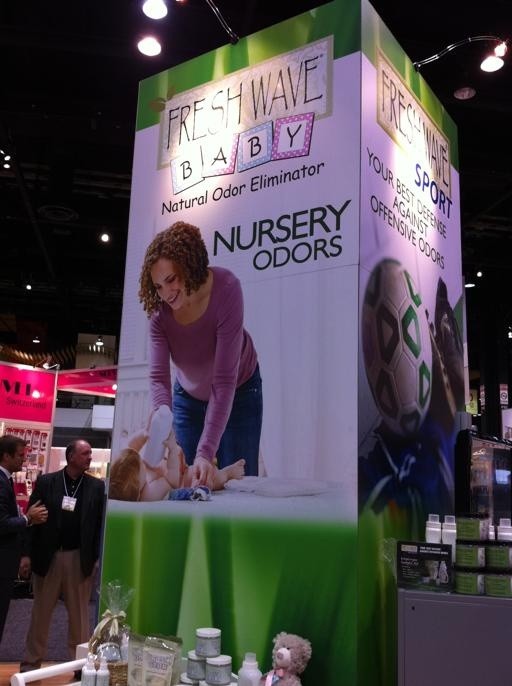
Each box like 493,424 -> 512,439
58,543 -> 81,551
181,375 -> 258,401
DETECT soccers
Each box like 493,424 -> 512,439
362,259 -> 433,438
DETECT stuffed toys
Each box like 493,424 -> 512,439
257,631 -> 313,686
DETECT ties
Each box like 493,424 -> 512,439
8,476 -> 13,487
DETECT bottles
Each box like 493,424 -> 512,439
80,656 -> 96,686
96,657 -> 111,686
424,513 -> 512,563
143,405 -> 173,468
237,652 -> 263,686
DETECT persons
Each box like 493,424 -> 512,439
137,220 -> 264,477
109,430 -> 247,503
17,439 -> 107,680
0,435 -> 49,651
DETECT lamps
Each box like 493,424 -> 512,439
414,32 -> 509,76
